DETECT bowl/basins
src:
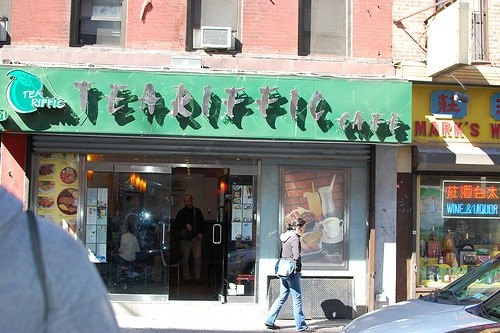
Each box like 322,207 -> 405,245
60,167 -> 77,185
57,187 -> 78,215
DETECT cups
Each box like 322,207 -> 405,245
68,219 -> 77,235
322,217 -> 343,239
307,192 -> 322,223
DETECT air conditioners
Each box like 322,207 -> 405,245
0,20 -> 7,42
201,26 -> 231,49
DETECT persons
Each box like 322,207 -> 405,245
175,194 -> 207,284
266,221 -> 315,332
0,185 -> 118,333
111,222 -> 140,286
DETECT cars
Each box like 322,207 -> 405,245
228,247 -> 257,291
338,250 -> 500,333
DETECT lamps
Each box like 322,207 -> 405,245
449,73 -> 469,103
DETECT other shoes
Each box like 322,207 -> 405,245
300,327 -> 312,332
265,323 -> 280,329
195,279 -> 202,284
185,279 -> 194,284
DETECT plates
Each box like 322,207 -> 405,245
38,198 -> 55,209
39,181 -> 56,193
298,222 -> 322,256
322,229 -> 343,243
40,152 -> 56,176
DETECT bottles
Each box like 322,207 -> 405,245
438,249 -> 444,264
89,199 -> 105,219
428,225 -> 440,240
451,254 -> 458,268
419,266 -> 465,286
420,232 -> 426,258
443,229 -> 459,249
317,186 -> 336,218
475,255 -> 494,284
460,232 -> 476,251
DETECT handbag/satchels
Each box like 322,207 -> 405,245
175,229 -> 190,240
275,259 -> 294,280
